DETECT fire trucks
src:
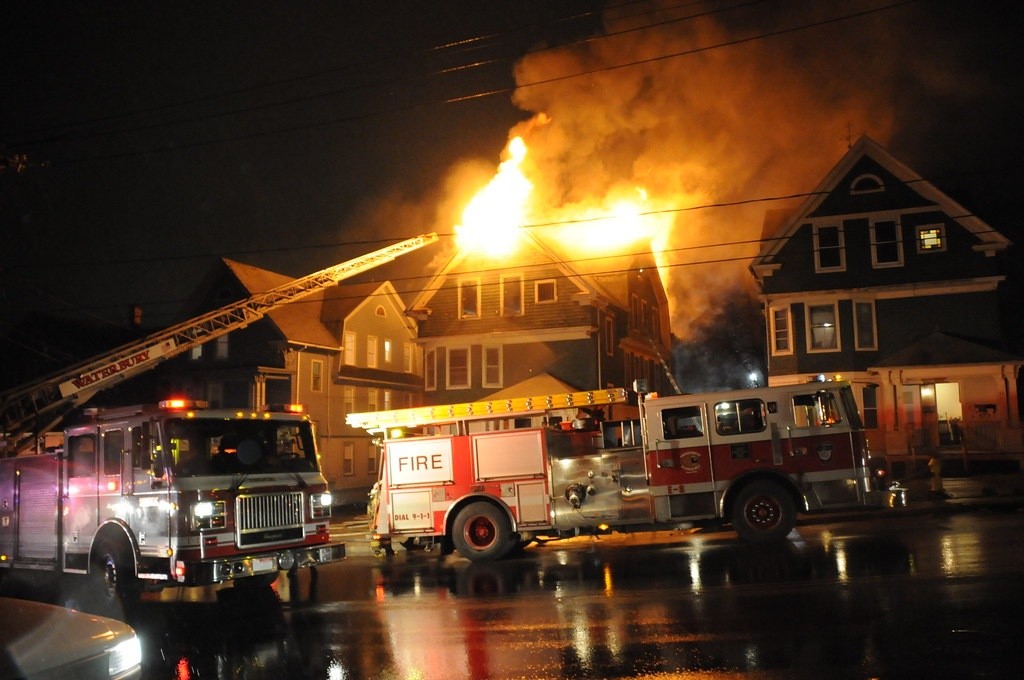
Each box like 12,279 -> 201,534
350,373 -> 910,566
0,233 -> 444,626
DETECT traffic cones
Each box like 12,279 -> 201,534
178,656 -> 196,680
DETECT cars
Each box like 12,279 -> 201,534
0,594 -> 141,679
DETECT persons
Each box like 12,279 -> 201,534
741,408 -> 759,431
367,481 -> 396,556
